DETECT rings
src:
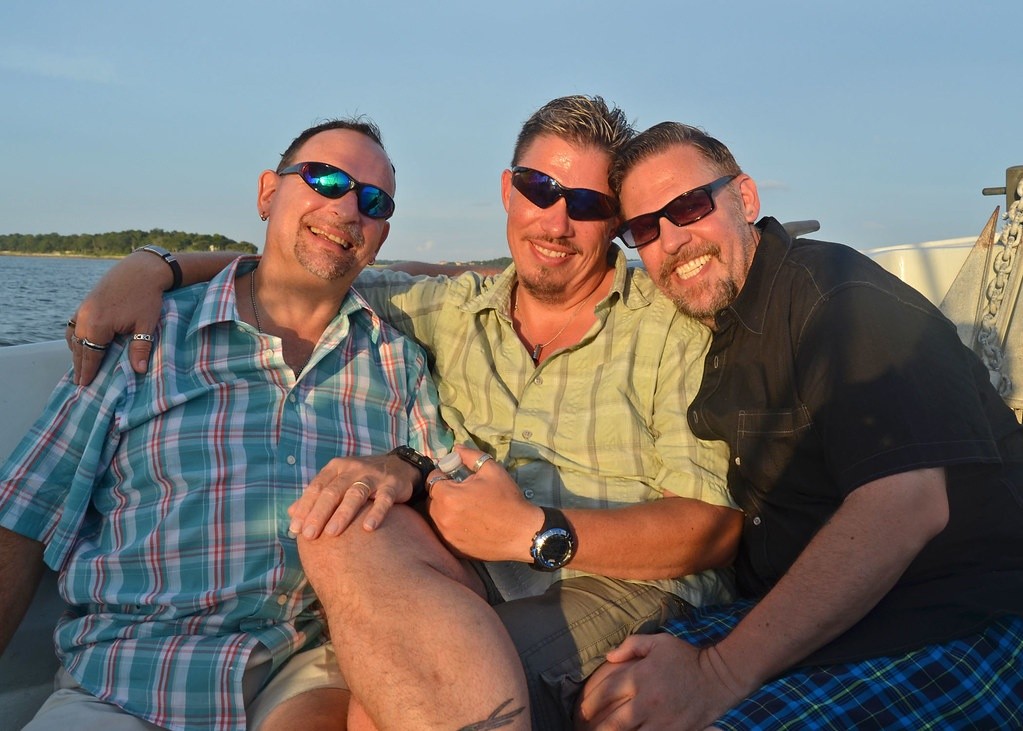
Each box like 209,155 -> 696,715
129,333 -> 154,342
82,338 -> 108,351
67,319 -> 76,327
427,475 -> 453,500
351,481 -> 372,497
472,454 -> 494,472
71,334 -> 83,344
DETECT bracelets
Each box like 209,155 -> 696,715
132,245 -> 182,292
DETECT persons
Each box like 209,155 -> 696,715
65,95 -> 745,731
580,121 -> 1022,731
1,119 -> 455,731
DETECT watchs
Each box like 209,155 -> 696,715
528,506 -> 575,572
387,445 -> 436,506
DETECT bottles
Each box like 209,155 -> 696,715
432,445 -> 546,603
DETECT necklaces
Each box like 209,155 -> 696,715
515,285 -> 594,361
251,268 -> 313,382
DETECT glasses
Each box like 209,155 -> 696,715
617,175 -> 738,249
279,161 -> 396,221
512,164 -> 619,223
